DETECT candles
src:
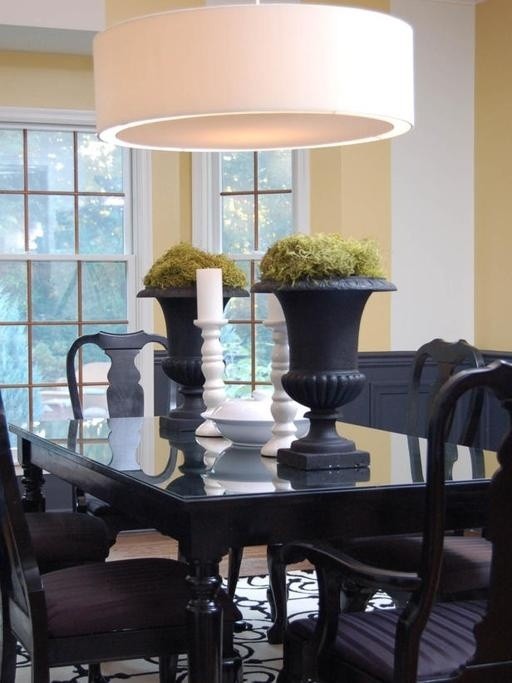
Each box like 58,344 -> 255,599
194,267 -> 230,314
260,292 -> 300,320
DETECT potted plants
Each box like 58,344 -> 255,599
249,228 -> 399,473
136,239 -> 252,434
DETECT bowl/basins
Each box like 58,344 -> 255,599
202,392 -> 310,446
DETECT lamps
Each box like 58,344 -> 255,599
92,0 -> 415,152
